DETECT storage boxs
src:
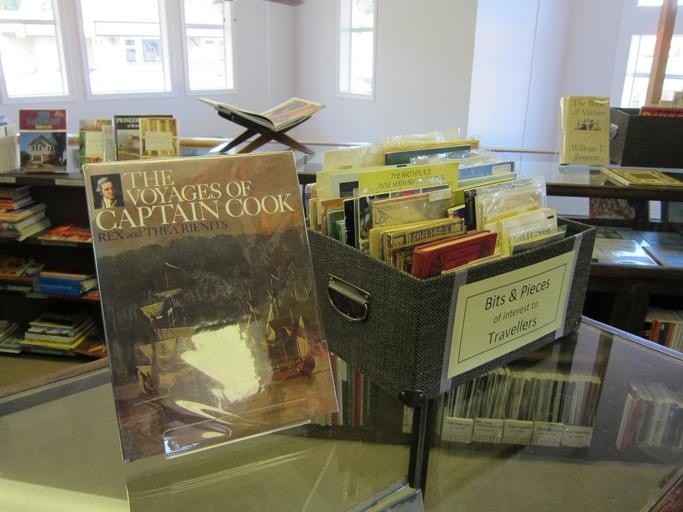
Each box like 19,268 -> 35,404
608,106 -> 683,168
303,215 -> 598,408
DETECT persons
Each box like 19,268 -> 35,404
96,176 -> 122,209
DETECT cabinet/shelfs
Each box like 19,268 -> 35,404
304,148 -> 683,464
0,135 -> 373,360
0,314 -> 682,512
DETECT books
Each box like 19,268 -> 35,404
445,363 -> 603,427
0,184 -> 92,243
1,310 -> 109,359
18,108 -> 69,174
313,351 -> 415,435
0,254 -> 98,296
197,95 -> 327,132
77,113 -> 180,170
307,129 -> 567,280
346,476 -> 427,512
559,95 -> 611,166
614,380 -> 683,454
599,167 -> 683,188
81,149 -> 339,462
642,307 -> 683,353
